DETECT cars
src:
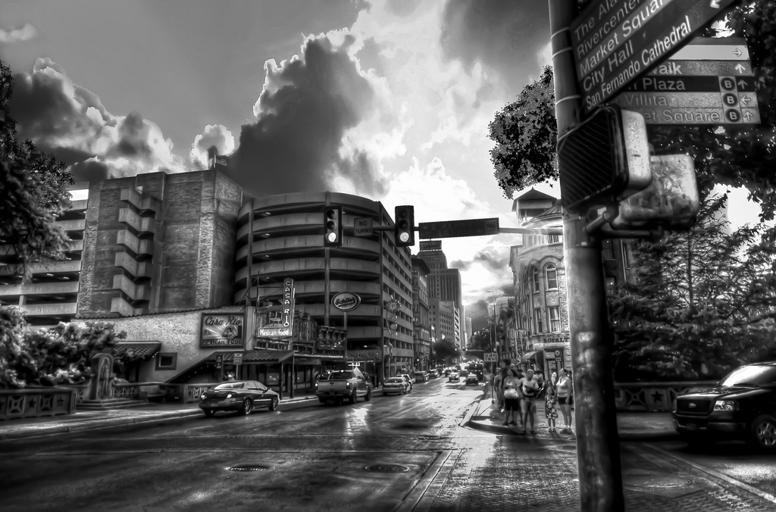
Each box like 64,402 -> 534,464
199,381 -> 279,417
382,374 -> 413,396
672,360 -> 776,456
415,360 -> 483,386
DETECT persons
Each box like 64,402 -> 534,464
493,359 -> 573,434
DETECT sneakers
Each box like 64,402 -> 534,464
549,428 -> 571,434
502,420 -> 537,434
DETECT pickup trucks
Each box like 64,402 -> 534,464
315,370 -> 372,404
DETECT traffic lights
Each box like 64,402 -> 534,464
395,205 -> 415,247
323,206 -> 343,249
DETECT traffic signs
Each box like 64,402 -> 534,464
569,0 -> 761,125
